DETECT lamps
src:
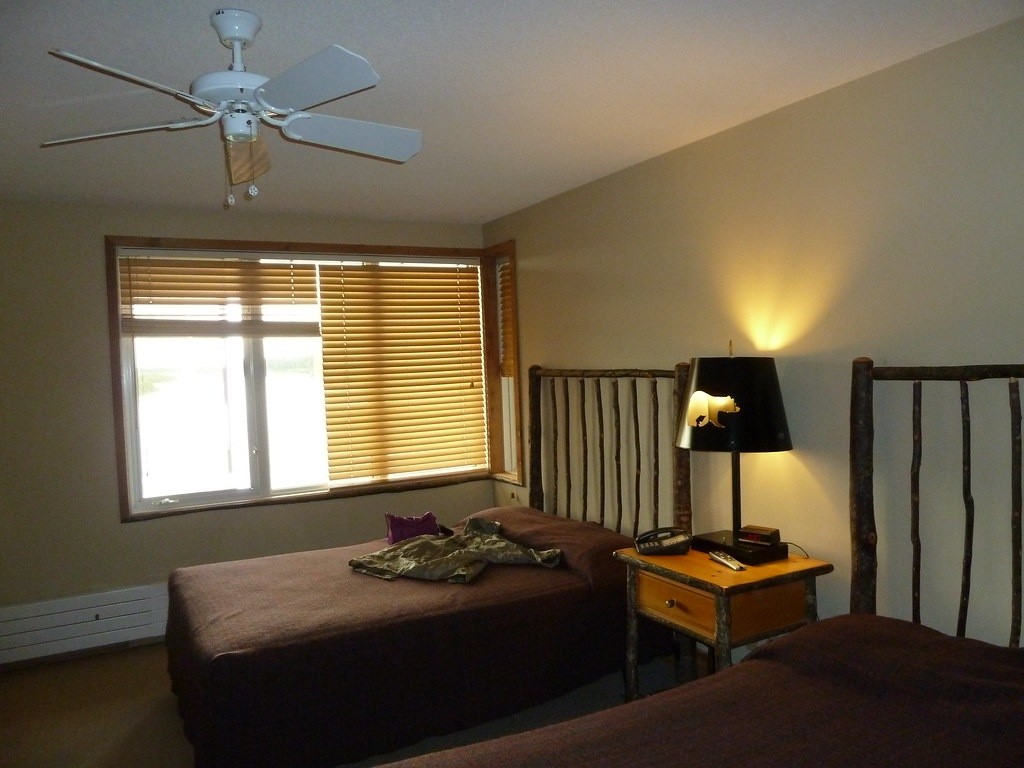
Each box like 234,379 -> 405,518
676,339 -> 809,566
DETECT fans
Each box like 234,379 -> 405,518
39,9 -> 421,206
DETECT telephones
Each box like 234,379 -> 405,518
634,526 -> 693,556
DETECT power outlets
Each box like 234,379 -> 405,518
746,639 -> 765,650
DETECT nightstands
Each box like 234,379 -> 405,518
613,547 -> 833,703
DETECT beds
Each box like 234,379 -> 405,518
166,362 -> 688,768
379,356 -> 1024,768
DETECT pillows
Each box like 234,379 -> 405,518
740,614 -> 1024,742
456,505 -> 633,586
